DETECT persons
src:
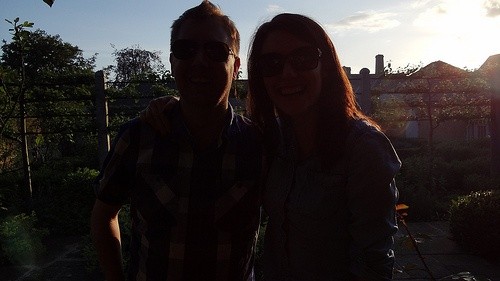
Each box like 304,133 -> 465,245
91,0 -> 268,281
145,12 -> 404,280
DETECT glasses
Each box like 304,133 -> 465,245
172,38 -> 234,63
255,46 -> 324,78
